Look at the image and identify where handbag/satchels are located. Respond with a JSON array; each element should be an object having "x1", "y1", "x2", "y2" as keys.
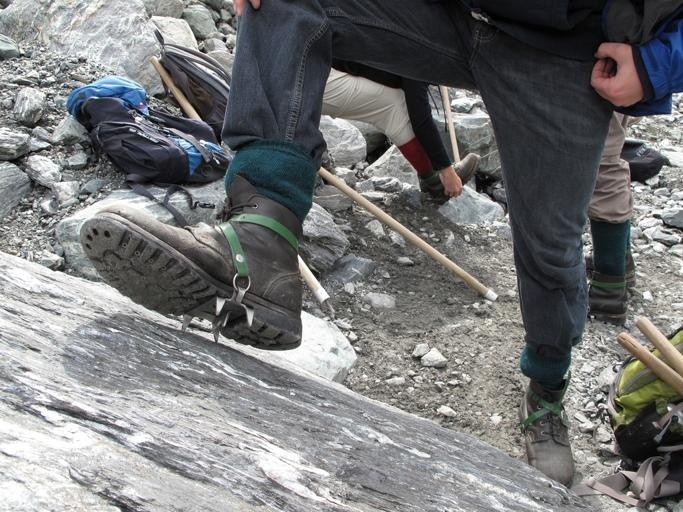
[{"x1": 620, "y1": 140, "x2": 665, "y2": 180}]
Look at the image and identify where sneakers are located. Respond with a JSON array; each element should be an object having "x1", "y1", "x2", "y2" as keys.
[{"x1": 419, "y1": 152, "x2": 481, "y2": 207}]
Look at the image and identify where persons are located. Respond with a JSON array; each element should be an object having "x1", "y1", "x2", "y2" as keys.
[
  {"x1": 79, "y1": 0, "x2": 683, "y2": 487},
  {"x1": 589, "y1": 110, "x2": 635, "y2": 326},
  {"x1": 321, "y1": 59, "x2": 482, "y2": 206}
]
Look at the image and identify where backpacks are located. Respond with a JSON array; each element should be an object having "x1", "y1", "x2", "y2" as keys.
[
  {"x1": 67, "y1": 75, "x2": 229, "y2": 186},
  {"x1": 607, "y1": 326, "x2": 683, "y2": 467},
  {"x1": 153, "y1": 29, "x2": 232, "y2": 146}
]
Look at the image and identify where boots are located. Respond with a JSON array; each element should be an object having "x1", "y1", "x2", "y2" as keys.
[
  {"x1": 585, "y1": 254, "x2": 636, "y2": 326},
  {"x1": 80, "y1": 173, "x2": 301, "y2": 350},
  {"x1": 518, "y1": 379, "x2": 573, "y2": 485}
]
